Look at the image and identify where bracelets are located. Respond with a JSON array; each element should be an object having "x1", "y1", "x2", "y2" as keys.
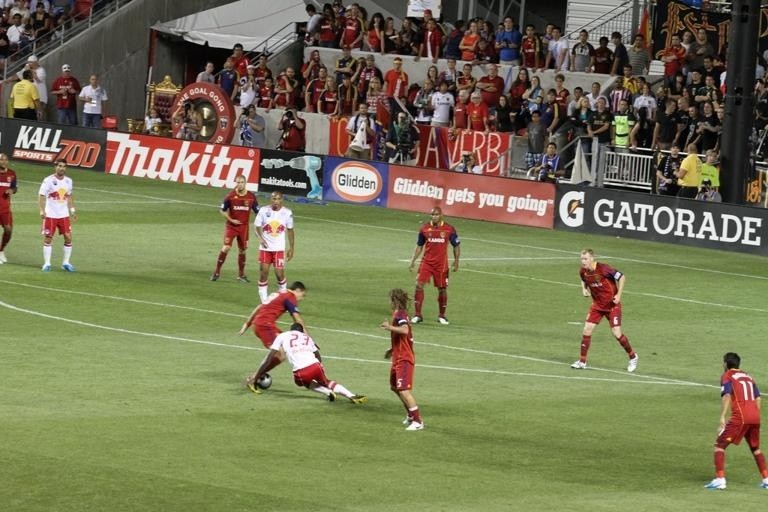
[{"x1": 70, "y1": 208, "x2": 76, "y2": 213}]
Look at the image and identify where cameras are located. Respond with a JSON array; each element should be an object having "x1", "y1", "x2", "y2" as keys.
[
  {"x1": 285, "y1": 111, "x2": 293, "y2": 119},
  {"x1": 241, "y1": 107, "x2": 250, "y2": 117},
  {"x1": 184, "y1": 103, "x2": 191, "y2": 112}
]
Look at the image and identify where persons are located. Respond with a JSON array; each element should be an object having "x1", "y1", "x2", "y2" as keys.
[
  {"x1": 0, "y1": 153, "x2": 17, "y2": 264},
  {"x1": 571, "y1": 249, "x2": 638, "y2": 372},
  {"x1": 303, "y1": 1, "x2": 497, "y2": 66},
  {"x1": 38, "y1": 158, "x2": 79, "y2": 272},
  {"x1": 704, "y1": 352, "x2": 768, "y2": 489},
  {"x1": 254, "y1": 190, "x2": 294, "y2": 304},
  {"x1": 239, "y1": 281, "x2": 306, "y2": 394},
  {"x1": 407, "y1": 206, "x2": 460, "y2": 324},
  {"x1": 233, "y1": 104, "x2": 266, "y2": 149},
  {"x1": 217, "y1": 43, "x2": 301, "y2": 114},
  {"x1": 449, "y1": 153, "x2": 482, "y2": 174},
  {"x1": 494, "y1": 67, "x2": 614, "y2": 168},
  {"x1": 1, "y1": 1, "x2": 112, "y2": 61},
  {"x1": 49, "y1": 64, "x2": 82, "y2": 125},
  {"x1": 172, "y1": 100, "x2": 204, "y2": 142},
  {"x1": 145, "y1": 109, "x2": 162, "y2": 136},
  {"x1": 79, "y1": 73, "x2": 108, "y2": 130},
  {"x1": 277, "y1": 106, "x2": 306, "y2": 153},
  {"x1": 209, "y1": 175, "x2": 259, "y2": 283},
  {"x1": 608, "y1": 27, "x2": 768, "y2": 202},
  {"x1": 386, "y1": 111, "x2": 420, "y2": 166},
  {"x1": 406, "y1": 58, "x2": 505, "y2": 132},
  {"x1": 345, "y1": 103, "x2": 376, "y2": 161},
  {"x1": 495, "y1": 17, "x2": 615, "y2": 75},
  {"x1": 196, "y1": 62, "x2": 215, "y2": 85},
  {"x1": 534, "y1": 142, "x2": 566, "y2": 183},
  {"x1": 251, "y1": 322, "x2": 368, "y2": 404},
  {"x1": 301, "y1": 44, "x2": 409, "y2": 118},
  {"x1": 2, "y1": 55, "x2": 48, "y2": 121},
  {"x1": 10, "y1": 70, "x2": 45, "y2": 121},
  {"x1": 380, "y1": 288, "x2": 424, "y2": 431}
]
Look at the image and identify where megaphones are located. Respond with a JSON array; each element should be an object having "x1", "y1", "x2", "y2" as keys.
[{"x1": 349, "y1": 117, "x2": 366, "y2": 152}]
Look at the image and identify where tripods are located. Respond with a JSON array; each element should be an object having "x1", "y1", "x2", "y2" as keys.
[{"x1": 390, "y1": 148, "x2": 403, "y2": 165}]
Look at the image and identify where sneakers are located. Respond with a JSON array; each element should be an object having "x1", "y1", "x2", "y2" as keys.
[
  {"x1": 705, "y1": 477, "x2": 726, "y2": 489},
  {"x1": 41, "y1": 265, "x2": 51, "y2": 271},
  {"x1": 61, "y1": 263, "x2": 75, "y2": 271},
  {"x1": 402, "y1": 415, "x2": 423, "y2": 430},
  {"x1": 411, "y1": 317, "x2": 450, "y2": 325},
  {"x1": 349, "y1": 394, "x2": 368, "y2": 403},
  {"x1": 0, "y1": 251, "x2": 7, "y2": 264},
  {"x1": 210, "y1": 273, "x2": 219, "y2": 281},
  {"x1": 238, "y1": 276, "x2": 248, "y2": 282},
  {"x1": 628, "y1": 353, "x2": 638, "y2": 372},
  {"x1": 246, "y1": 382, "x2": 262, "y2": 393},
  {"x1": 571, "y1": 360, "x2": 586, "y2": 369}
]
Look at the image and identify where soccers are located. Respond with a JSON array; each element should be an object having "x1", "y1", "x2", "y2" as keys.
[{"x1": 257, "y1": 374, "x2": 272, "y2": 389}]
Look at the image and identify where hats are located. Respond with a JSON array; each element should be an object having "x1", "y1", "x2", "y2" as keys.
[
  {"x1": 62, "y1": 64, "x2": 71, "y2": 71},
  {"x1": 28, "y1": 56, "x2": 37, "y2": 62}
]
